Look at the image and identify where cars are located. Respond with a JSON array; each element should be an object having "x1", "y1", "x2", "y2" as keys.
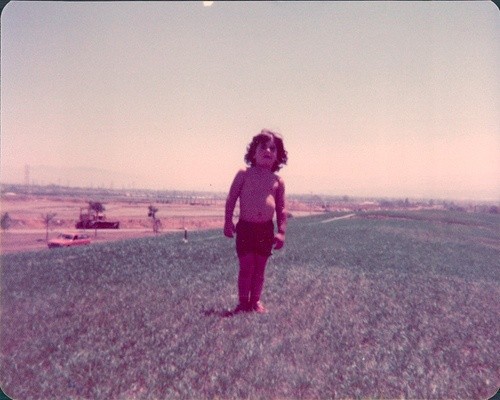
[{"x1": 47, "y1": 232, "x2": 92, "y2": 249}]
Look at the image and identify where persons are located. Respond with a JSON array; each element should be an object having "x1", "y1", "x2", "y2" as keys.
[{"x1": 224, "y1": 128, "x2": 288, "y2": 314}]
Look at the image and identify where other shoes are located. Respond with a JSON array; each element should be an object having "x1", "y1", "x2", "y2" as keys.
[
  {"x1": 249, "y1": 301, "x2": 267, "y2": 313},
  {"x1": 235, "y1": 305, "x2": 248, "y2": 313}
]
